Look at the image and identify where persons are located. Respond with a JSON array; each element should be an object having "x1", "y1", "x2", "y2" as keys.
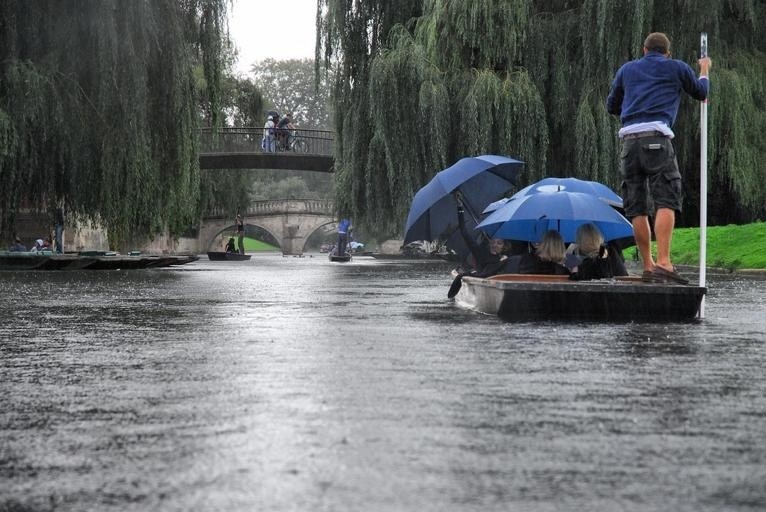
[
  {"x1": 235, "y1": 214, "x2": 245, "y2": 255},
  {"x1": 454, "y1": 190, "x2": 628, "y2": 281},
  {"x1": 336, "y1": 218, "x2": 354, "y2": 256},
  {"x1": 9, "y1": 234, "x2": 52, "y2": 252},
  {"x1": 224, "y1": 238, "x2": 236, "y2": 253},
  {"x1": 605, "y1": 32, "x2": 712, "y2": 285},
  {"x1": 261, "y1": 113, "x2": 296, "y2": 152}
]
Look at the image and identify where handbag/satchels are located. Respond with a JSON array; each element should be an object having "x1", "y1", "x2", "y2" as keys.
[{"x1": 267, "y1": 122, "x2": 274, "y2": 134}]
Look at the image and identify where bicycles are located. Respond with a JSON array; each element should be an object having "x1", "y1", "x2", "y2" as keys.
[{"x1": 268, "y1": 129, "x2": 311, "y2": 153}]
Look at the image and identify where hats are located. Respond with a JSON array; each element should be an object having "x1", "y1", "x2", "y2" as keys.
[{"x1": 268, "y1": 116, "x2": 273, "y2": 120}]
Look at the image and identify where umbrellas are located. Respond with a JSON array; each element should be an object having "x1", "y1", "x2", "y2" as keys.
[
  {"x1": 473, "y1": 177, "x2": 633, "y2": 244},
  {"x1": 403, "y1": 155, "x2": 526, "y2": 247}
]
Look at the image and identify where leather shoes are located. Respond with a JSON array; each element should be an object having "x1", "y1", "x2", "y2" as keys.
[{"x1": 642, "y1": 265, "x2": 689, "y2": 285}]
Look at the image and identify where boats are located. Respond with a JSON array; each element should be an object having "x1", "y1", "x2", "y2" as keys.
[
  {"x1": 444, "y1": 253, "x2": 709, "y2": 323},
  {"x1": 317, "y1": 240, "x2": 365, "y2": 263},
  {"x1": 0, "y1": 249, "x2": 202, "y2": 270},
  {"x1": 205, "y1": 250, "x2": 252, "y2": 261}
]
